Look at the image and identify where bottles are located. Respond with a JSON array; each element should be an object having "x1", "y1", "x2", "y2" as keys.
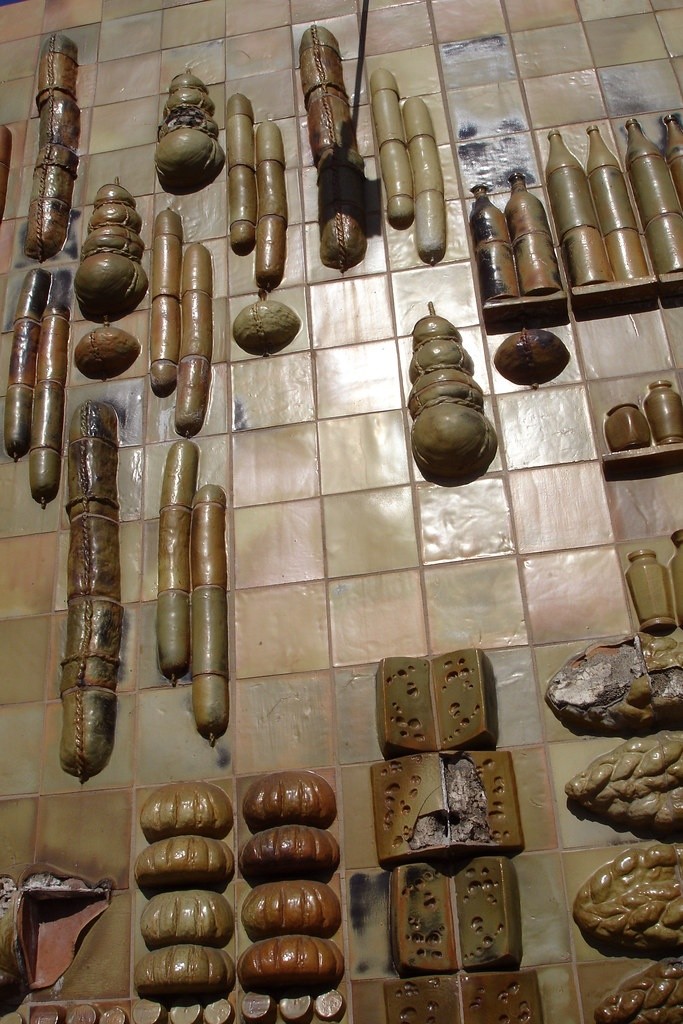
[
  {"x1": 585, "y1": 126, "x2": 648, "y2": 281},
  {"x1": 626, "y1": 549, "x2": 675, "y2": 633},
  {"x1": 669, "y1": 530, "x2": 683, "y2": 626},
  {"x1": 625, "y1": 119, "x2": 683, "y2": 274},
  {"x1": 504, "y1": 173, "x2": 562, "y2": 296},
  {"x1": 664, "y1": 116, "x2": 683, "y2": 210},
  {"x1": 643, "y1": 381, "x2": 683, "y2": 445},
  {"x1": 471, "y1": 186, "x2": 519, "y2": 301},
  {"x1": 543, "y1": 129, "x2": 612, "y2": 287}
]
